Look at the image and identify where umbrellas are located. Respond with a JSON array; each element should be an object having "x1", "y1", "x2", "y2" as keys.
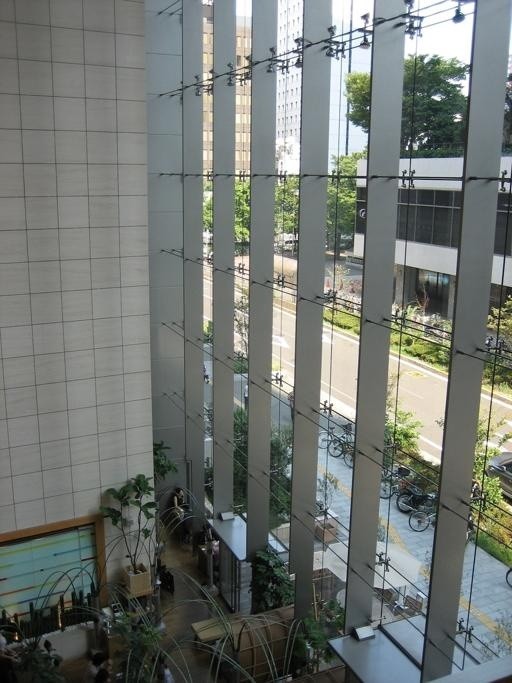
[{"x1": 325, "y1": 539, "x2": 428, "y2": 590}]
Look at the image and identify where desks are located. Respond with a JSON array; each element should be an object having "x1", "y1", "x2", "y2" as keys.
[
  {"x1": 198, "y1": 545, "x2": 206, "y2": 575},
  {"x1": 191, "y1": 612, "x2": 243, "y2": 654}
]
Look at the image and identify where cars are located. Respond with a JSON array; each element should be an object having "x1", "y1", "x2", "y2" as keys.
[{"x1": 481, "y1": 451, "x2": 512, "y2": 500}]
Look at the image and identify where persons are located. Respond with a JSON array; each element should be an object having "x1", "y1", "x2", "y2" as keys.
[
  {"x1": 287, "y1": 390, "x2": 294, "y2": 421},
  {"x1": 470, "y1": 481, "x2": 485, "y2": 500},
  {"x1": 203, "y1": 364, "x2": 210, "y2": 385},
  {"x1": 466, "y1": 513, "x2": 474, "y2": 541},
  {"x1": 173, "y1": 488, "x2": 189, "y2": 516},
  {"x1": 243, "y1": 380, "x2": 249, "y2": 412}
]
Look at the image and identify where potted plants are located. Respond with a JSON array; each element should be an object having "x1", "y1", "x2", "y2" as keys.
[{"x1": 97, "y1": 474, "x2": 157, "y2": 597}]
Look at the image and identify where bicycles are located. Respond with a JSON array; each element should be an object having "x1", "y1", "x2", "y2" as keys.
[
  {"x1": 506, "y1": 566, "x2": 512, "y2": 588},
  {"x1": 204, "y1": 374, "x2": 210, "y2": 384},
  {"x1": 318, "y1": 422, "x2": 439, "y2": 530}
]
